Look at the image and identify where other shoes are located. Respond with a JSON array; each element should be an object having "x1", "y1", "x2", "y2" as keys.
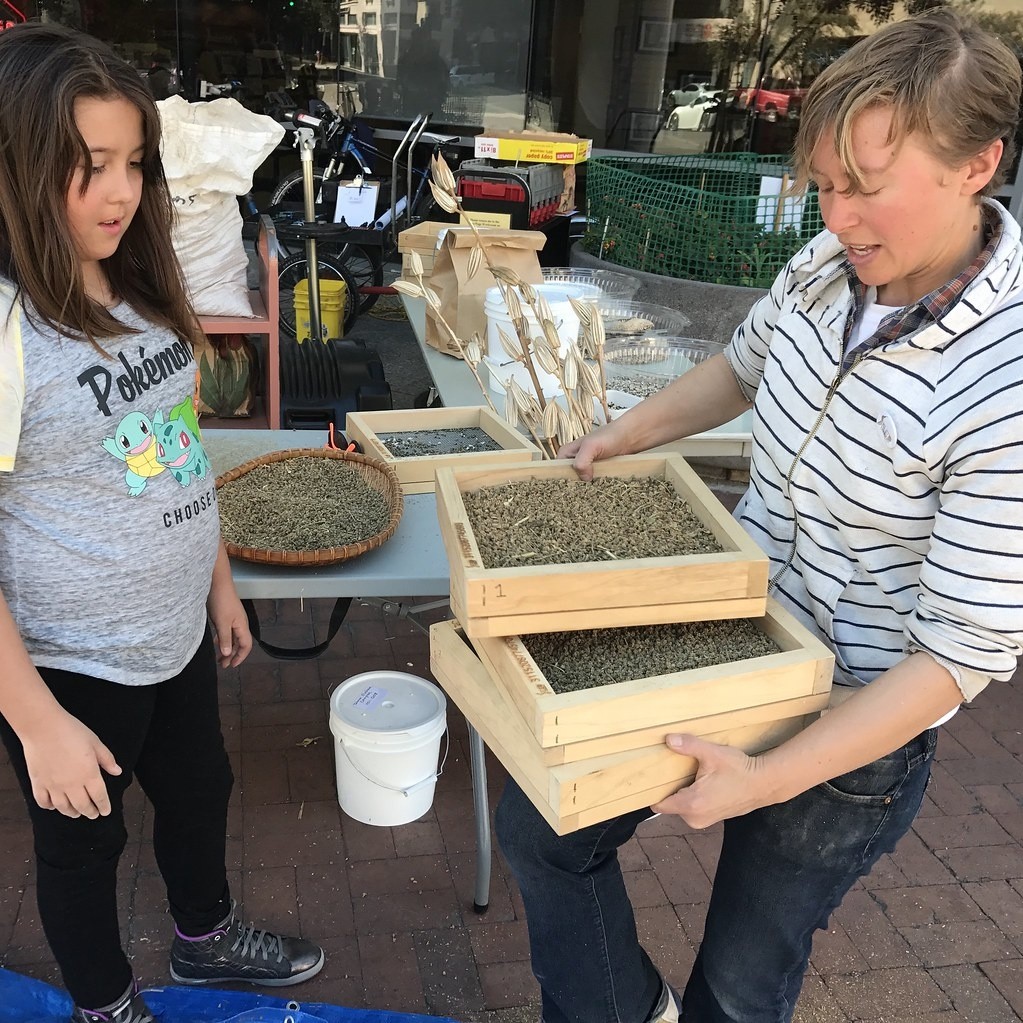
[
  {"x1": 169, "y1": 898, "x2": 325, "y2": 987},
  {"x1": 70, "y1": 963, "x2": 157, "y2": 1023}
]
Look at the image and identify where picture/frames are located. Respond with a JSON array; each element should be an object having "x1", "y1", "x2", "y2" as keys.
[
  {"x1": 624, "y1": 110, "x2": 661, "y2": 144},
  {"x1": 633, "y1": 16, "x2": 682, "y2": 56}
]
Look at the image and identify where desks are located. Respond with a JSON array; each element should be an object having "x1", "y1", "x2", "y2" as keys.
[
  {"x1": 201, "y1": 428, "x2": 493, "y2": 914},
  {"x1": 395, "y1": 285, "x2": 756, "y2": 461}
]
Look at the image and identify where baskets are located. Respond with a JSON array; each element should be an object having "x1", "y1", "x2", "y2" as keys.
[{"x1": 213, "y1": 447, "x2": 404, "y2": 567}]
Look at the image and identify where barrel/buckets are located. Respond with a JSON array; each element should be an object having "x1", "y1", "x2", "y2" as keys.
[
  {"x1": 483, "y1": 285, "x2": 584, "y2": 398},
  {"x1": 328, "y1": 671, "x2": 448, "y2": 827},
  {"x1": 293, "y1": 279, "x2": 347, "y2": 345}
]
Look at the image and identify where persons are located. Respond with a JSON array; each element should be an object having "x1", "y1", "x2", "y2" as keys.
[
  {"x1": 494, "y1": 14, "x2": 1023, "y2": 1023},
  {"x1": 0, "y1": 23, "x2": 325, "y2": 1023}
]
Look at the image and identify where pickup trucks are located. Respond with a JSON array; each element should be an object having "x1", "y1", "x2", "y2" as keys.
[{"x1": 745, "y1": 74, "x2": 811, "y2": 124}]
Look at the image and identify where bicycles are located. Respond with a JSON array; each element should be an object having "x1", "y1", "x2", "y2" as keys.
[{"x1": 241, "y1": 79, "x2": 580, "y2": 341}]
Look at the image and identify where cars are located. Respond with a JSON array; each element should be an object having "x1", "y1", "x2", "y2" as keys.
[
  {"x1": 448, "y1": 63, "x2": 498, "y2": 87},
  {"x1": 665, "y1": 90, "x2": 747, "y2": 133},
  {"x1": 666, "y1": 82, "x2": 724, "y2": 108}
]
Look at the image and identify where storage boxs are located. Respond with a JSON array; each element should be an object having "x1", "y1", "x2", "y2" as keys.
[
  {"x1": 401, "y1": 274, "x2": 430, "y2": 287},
  {"x1": 343, "y1": 405, "x2": 543, "y2": 496},
  {"x1": 395, "y1": 222, "x2": 466, "y2": 254},
  {"x1": 432, "y1": 452, "x2": 769, "y2": 637},
  {"x1": 472, "y1": 129, "x2": 593, "y2": 164},
  {"x1": 402, "y1": 255, "x2": 433, "y2": 277},
  {"x1": 428, "y1": 619, "x2": 821, "y2": 836},
  {"x1": 454, "y1": 157, "x2": 565, "y2": 207},
  {"x1": 293, "y1": 279, "x2": 347, "y2": 345},
  {"x1": 450, "y1": 599, "x2": 835, "y2": 767},
  {"x1": 458, "y1": 177, "x2": 560, "y2": 226}
]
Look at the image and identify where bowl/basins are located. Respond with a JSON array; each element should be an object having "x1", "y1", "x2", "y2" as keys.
[
  {"x1": 541, "y1": 267, "x2": 640, "y2": 301},
  {"x1": 595, "y1": 336, "x2": 729, "y2": 400},
  {"x1": 578, "y1": 300, "x2": 690, "y2": 365},
  {"x1": 592, "y1": 390, "x2": 644, "y2": 424}
]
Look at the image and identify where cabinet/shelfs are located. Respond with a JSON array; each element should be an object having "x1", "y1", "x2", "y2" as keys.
[{"x1": 190, "y1": 212, "x2": 280, "y2": 430}]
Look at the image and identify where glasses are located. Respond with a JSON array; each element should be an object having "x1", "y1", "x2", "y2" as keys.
[{"x1": 326, "y1": 423, "x2": 362, "y2": 453}]
